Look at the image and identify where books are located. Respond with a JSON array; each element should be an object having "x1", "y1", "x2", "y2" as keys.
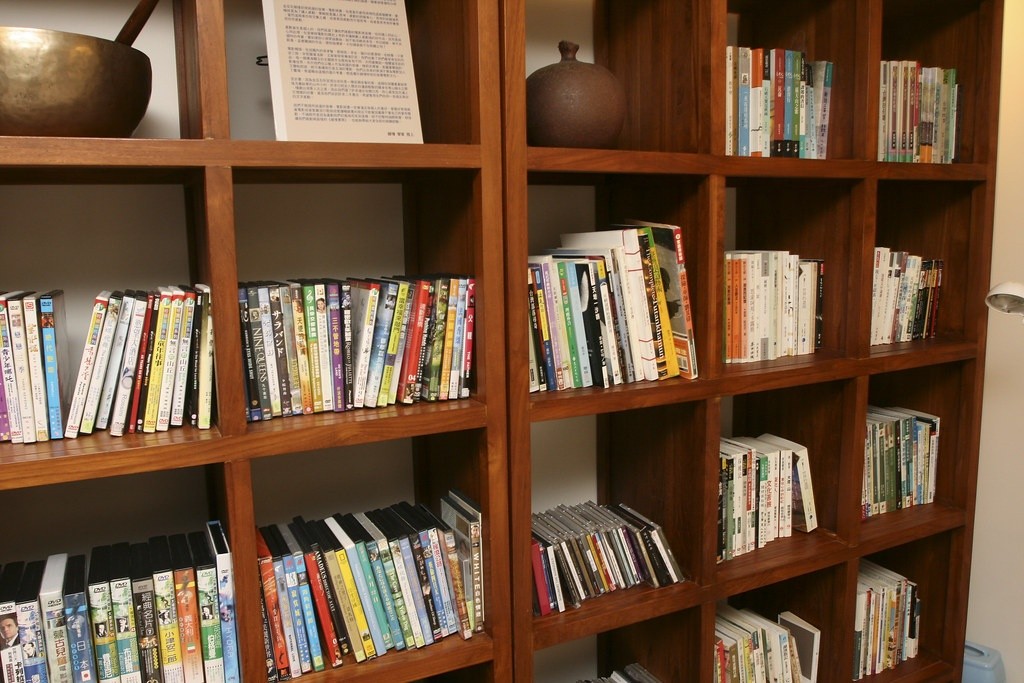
[
  {"x1": 722, "y1": 250, "x2": 825, "y2": 363},
  {"x1": 530, "y1": 218, "x2": 698, "y2": 392},
  {"x1": 576, "y1": 663, "x2": 663, "y2": 683},
  {"x1": 716, "y1": 433, "x2": 817, "y2": 563},
  {"x1": 878, "y1": 61, "x2": 958, "y2": 163},
  {"x1": 531, "y1": 500, "x2": 684, "y2": 616},
  {"x1": 0, "y1": 520, "x2": 241, "y2": 683},
  {"x1": 0, "y1": 284, "x2": 212, "y2": 444},
  {"x1": 255, "y1": 489, "x2": 485, "y2": 683},
  {"x1": 860, "y1": 406, "x2": 940, "y2": 519},
  {"x1": 870, "y1": 247, "x2": 944, "y2": 346},
  {"x1": 237, "y1": 274, "x2": 476, "y2": 422},
  {"x1": 714, "y1": 602, "x2": 820, "y2": 683},
  {"x1": 726, "y1": 46, "x2": 833, "y2": 159},
  {"x1": 852, "y1": 562, "x2": 921, "y2": 681}
]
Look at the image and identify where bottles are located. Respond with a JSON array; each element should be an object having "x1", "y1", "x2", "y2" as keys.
[{"x1": 526, "y1": 38, "x2": 627, "y2": 148}]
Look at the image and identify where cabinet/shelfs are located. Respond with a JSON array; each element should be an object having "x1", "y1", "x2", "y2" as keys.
[{"x1": 1, "y1": 0, "x2": 1002, "y2": 683}]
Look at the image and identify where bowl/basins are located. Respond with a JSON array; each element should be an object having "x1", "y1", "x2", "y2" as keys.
[{"x1": 0, "y1": 27, "x2": 152, "y2": 138}]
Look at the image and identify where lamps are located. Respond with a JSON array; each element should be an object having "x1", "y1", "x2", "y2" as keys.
[{"x1": 984, "y1": 282, "x2": 1024, "y2": 316}]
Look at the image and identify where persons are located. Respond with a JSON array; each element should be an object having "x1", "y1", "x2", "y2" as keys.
[
  {"x1": 471, "y1": 525, "x2": 479, "y2": 538},
  {"x1": 293, "y1": 299, "x2": 302, "y2": 312},
  {"x1": 317, "y1": 298, "x2": 325, "y2": 312},
  {"x1": 265, "y1": 658, "x2": 276, "y2": 677},
  {"x1": 0, "y1": 616, "x2": 20, "y2": 649}
]
